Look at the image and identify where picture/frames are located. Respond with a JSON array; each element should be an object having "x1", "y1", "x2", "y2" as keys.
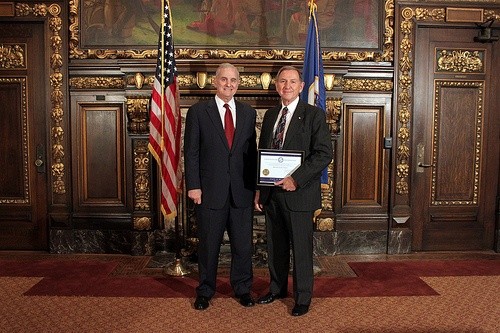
[
  {"x1": 256, "y1": 149, "x2": 305, "y2": 187},
  {"x1": 69, "y1": 0, "x2": 395, "y2": 60}
]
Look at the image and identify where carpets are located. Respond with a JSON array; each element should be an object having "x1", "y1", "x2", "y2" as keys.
[{"x1": 0, "y1": 253, "x2": 500, "y2": 297}]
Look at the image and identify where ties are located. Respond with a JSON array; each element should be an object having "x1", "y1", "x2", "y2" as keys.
[
  {"x1": 273, "y1": 107, "x2": 289, "y2": 150},
  {"x1": 223, "y1": 103, "x2": 235, "y2": 151}
]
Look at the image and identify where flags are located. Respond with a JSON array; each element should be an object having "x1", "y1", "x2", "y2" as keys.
[
  {"x1": 302, "y1": 0, "x2": 329, "y2": 185},
  {"x1": 148, "y1": 0, "x2": 183, "y2": 222}
]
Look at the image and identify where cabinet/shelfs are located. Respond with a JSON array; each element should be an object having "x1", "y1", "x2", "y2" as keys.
[
  {"x1": 69, "y1": 89, "x2": 133, "y2": 218},
  {"x1": 334, "y1": 91, "x2": 392, "y2": 216}
]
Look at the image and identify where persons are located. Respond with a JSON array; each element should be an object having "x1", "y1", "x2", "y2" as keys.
[
  {"x1": 253, "y1": 65, "x2": 334, "y2": 316},
  {"x1": 182, "y1": 62, "x2": 259, "y2": 311}
]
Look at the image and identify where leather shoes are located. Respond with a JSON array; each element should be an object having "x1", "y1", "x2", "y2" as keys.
[
  {"x1": 194, "y1": 296, "x2": 209, "y2": 309},
  {"x1": 257, "y1": 292, "x2": 288, "y2": 304},
  {"x1": 234, "y1": 294, "x2": 254, "y2": 306},
  {"x1": 292, "y1": 300, "x2": 311, "y2": 316}
]
